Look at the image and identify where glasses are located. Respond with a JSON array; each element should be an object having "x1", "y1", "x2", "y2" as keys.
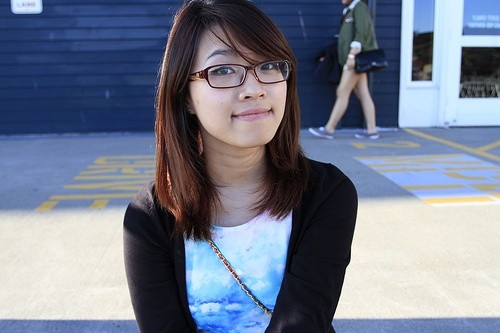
[{"x1": 190, "y1": 59, "x2": 292, "y2": 89}]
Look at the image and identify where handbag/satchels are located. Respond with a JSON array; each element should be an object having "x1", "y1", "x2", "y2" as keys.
[{"x1": 353, "y1": 48, "x2": 388, "y2": 74}]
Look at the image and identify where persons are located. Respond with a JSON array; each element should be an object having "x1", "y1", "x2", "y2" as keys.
[
  {"x1": 122, "y1": 0, "x2": 360, "y2": 333},
  {"x1": 308, "y1": 0, "x2": 381, "y2": 140}
]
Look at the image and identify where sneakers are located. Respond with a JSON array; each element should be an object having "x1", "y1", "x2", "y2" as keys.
[
  {"x1": 309, "y1": 127, "x2": 334, "y2": 139},
  {"x1": 354, "y1": 131, "x2": 381, "y2": 139}
]
